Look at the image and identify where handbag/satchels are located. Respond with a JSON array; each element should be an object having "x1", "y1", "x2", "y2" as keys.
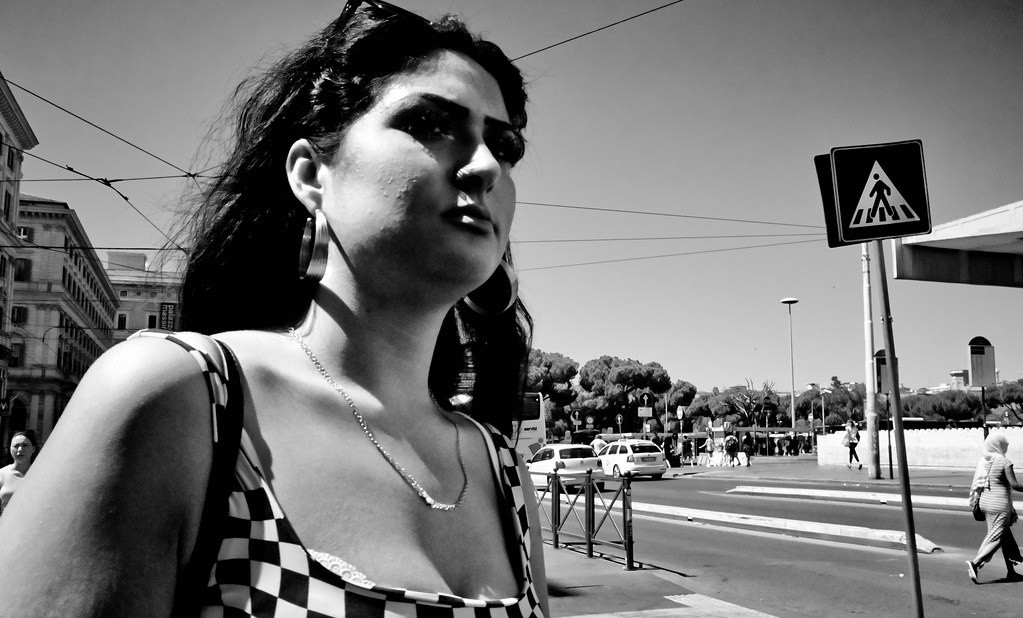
[
  {"x1": 972, "y1": 501, "x2": 986, "y2": 522},
  {"x1": 842, "y1": 427, "x2": 850, "y2": 447},
  {"x1": 856, "y1": 433, "x2": 860, "y2": 440},
  {"x1": 743, "y1": 444, "x2": 749, "y2": 452}
]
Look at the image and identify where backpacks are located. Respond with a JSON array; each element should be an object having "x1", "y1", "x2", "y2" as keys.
[{"x1": 725, "y1": 438, "x2": 738, "y2": 452}]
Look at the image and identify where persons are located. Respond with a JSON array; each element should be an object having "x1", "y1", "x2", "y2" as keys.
[
  {"x1": 742, "y1": 431, "x2": 753, "y2": 466},
  {"x1": 590, "y1": 435, "x2": 607, "y2": 455},
  {"x1": 642, "y1": 432, "x2": 695, "y2": 468},
  {"x1": 965, "y1": 433, "x2": 1023, "y2": 584},
  {"x1": 753, "y1": 436, "x2": 812, "y2": 456},
  {"x1": 844, "y1": 419, "x2": 863, "y2": 469},
  {"x1": 725, "y1": 432, "x2": 741, "y2": 467},
  {"x1": 0, "y1": 431, "x2": 38, "y2": 518},
  {"x1": 699, "y1": 433, "x2": 714, "y2": 468},
  {"x1": 1, "y1": 0, "x2": 550, "y2": 618}
]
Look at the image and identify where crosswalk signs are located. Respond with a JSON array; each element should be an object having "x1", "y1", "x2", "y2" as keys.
[{"x1": 830, "y1": 139, "x2": 932, "y2": 244}]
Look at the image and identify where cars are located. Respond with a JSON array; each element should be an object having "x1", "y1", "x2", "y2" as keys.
[
  {"x1": 524, "y1": 443, "x2": 605, "y2": 493},
  {"x1": 597, "y1": 438, "x2": 668, "y2": 481}
]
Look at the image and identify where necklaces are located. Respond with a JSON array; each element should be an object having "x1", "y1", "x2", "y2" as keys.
[{"x1": 285, "y1": 324, "x2": 469, "y2": 511}]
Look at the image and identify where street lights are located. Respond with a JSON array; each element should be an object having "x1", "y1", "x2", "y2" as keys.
[{"x1": 779, "y1": 297, "x2": 800, "y2": 433}]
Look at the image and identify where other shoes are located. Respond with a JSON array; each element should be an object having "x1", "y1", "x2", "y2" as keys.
[
  {"x1": 1006, "y1": 571, "x2": 1023, "y2": 581},
  {"x1": 745, "y1": 464, "x2": 750, "y2": 467},
  {"x1": 736, "y1": 463, "x2": 740, "y2": 466},
  {"x1": 847, "y1": 465, "x2": 852, "y2": 468},
  {"x1": 858, "y1": 464, "x2": 863, "y2": 470},
  {"x1": 965, "y1": 560, "x2": 980, "y2": 584}
]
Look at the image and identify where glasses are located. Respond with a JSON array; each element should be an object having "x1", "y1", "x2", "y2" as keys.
[{"x1": 332, "y1": 0, "x2": 442, "y2": 37}]
